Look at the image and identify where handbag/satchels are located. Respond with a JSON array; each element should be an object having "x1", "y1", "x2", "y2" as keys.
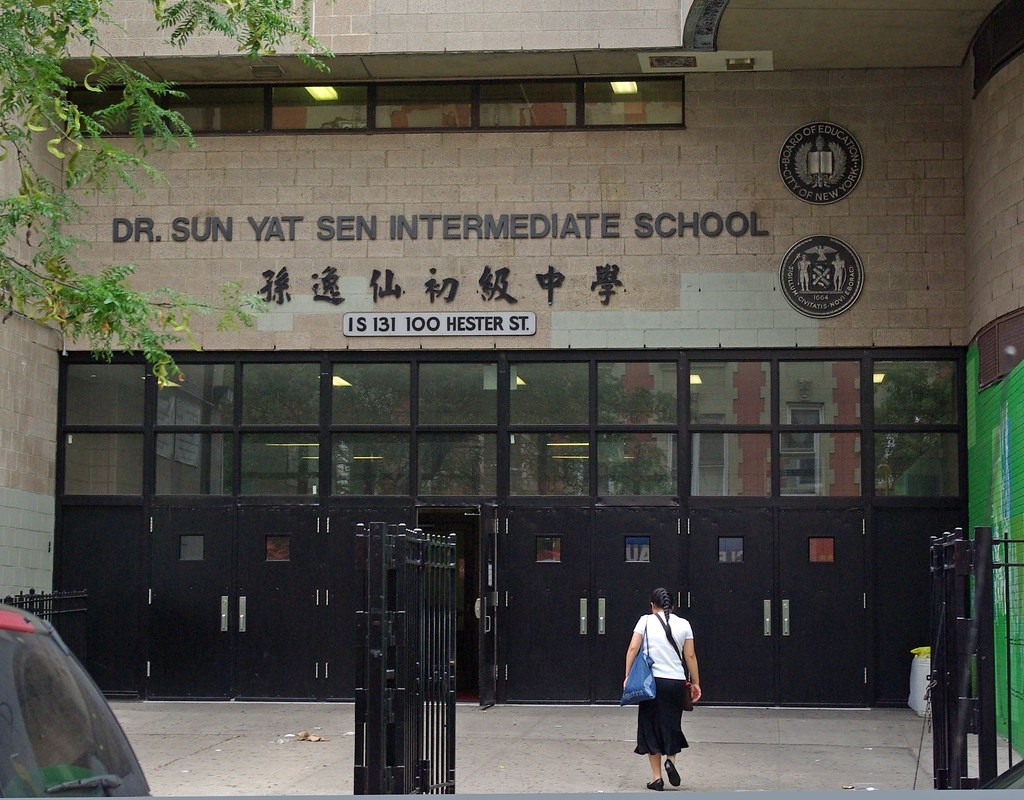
[
  {"x1": 620, "y1": 652, "x2": 655, "y2": 706},
  {"x1": 684, "y1": 684, "x2": 694, "y2": 712}
]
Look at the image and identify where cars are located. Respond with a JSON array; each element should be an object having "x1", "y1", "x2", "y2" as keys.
[{"x1": 0, "y1": 603, "x2": 150, "y2": 799}]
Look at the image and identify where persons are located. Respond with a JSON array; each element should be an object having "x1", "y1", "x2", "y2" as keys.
[{"x1": 623, "y1": 588, "x2": 701, "y2": 791}]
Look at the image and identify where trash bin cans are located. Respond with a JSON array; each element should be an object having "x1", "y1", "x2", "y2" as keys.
[{"x1": 907, "y1": 646, "x2": 932, "y2": 718}]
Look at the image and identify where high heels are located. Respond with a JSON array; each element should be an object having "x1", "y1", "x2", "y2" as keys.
[
  {"x1": 664, "y1": 759, "x2": 681, "y2": 786},
  {"x1": 647, "y1": 778, "x2": 664, "y2": 791}
]
